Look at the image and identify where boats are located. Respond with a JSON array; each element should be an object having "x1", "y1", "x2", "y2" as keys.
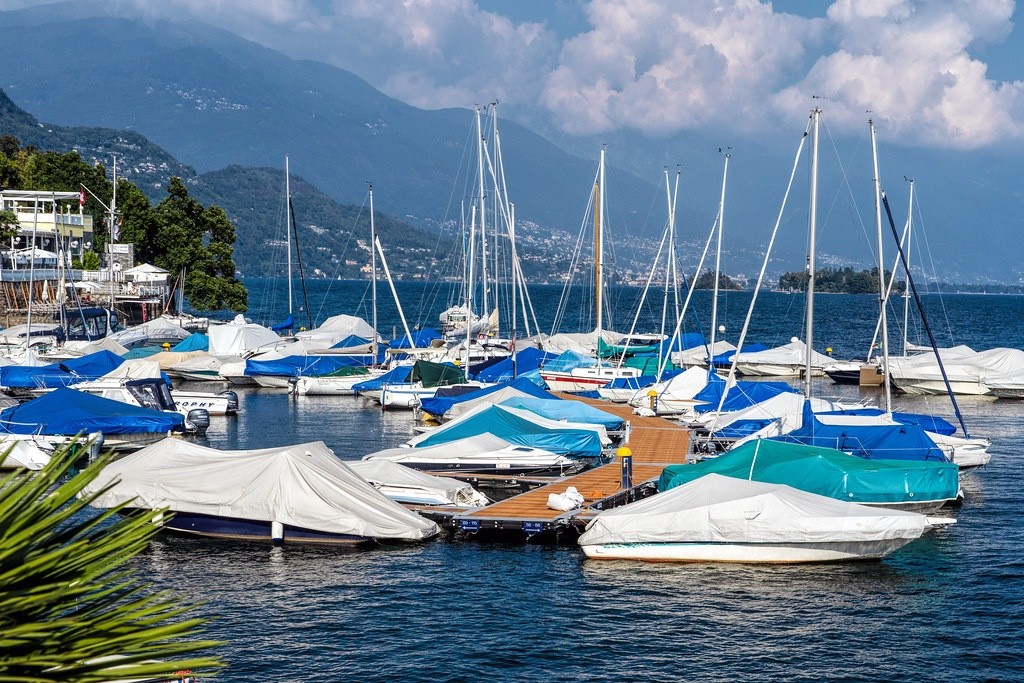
[
  {"x1": 0, "y1": 102, "x2": 993, "y2": 547},
  {"x1": 73, "y1": 435, "x2": 440, "y2": 547},
  {"x1": 656, "y1": 436, "x2": 966, "y2": 515},
  {"x1": 732, "y1": 409, "x2": 992, "y2": 471},
  {"x1": 0, "y1": 386, "x2": 186, "y2": 475},
  {"x1": 881, "y1": 343, "x2": 1024, "y2": 400},
  {"x1": 576, "y1": 472, "x2": 959, "y2": 563},
  {"x1": 357, "y1": 433, "x2": 584, "y2": 489},
  {"x1": 340, "y1": 455, "x2": 487, "y2": 527}
]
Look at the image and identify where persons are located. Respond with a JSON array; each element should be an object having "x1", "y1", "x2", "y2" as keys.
[
  {"x1": 54, "y1": 326, "x2": 67, "y2": 348},
  {"x1": 76, "y1": 290, "x2": 92, "y2": 303}
]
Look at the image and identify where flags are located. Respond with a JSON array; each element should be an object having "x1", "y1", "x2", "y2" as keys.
[{"x1": 80, "y1": 186, "x2": 87, "y2": 205}]
